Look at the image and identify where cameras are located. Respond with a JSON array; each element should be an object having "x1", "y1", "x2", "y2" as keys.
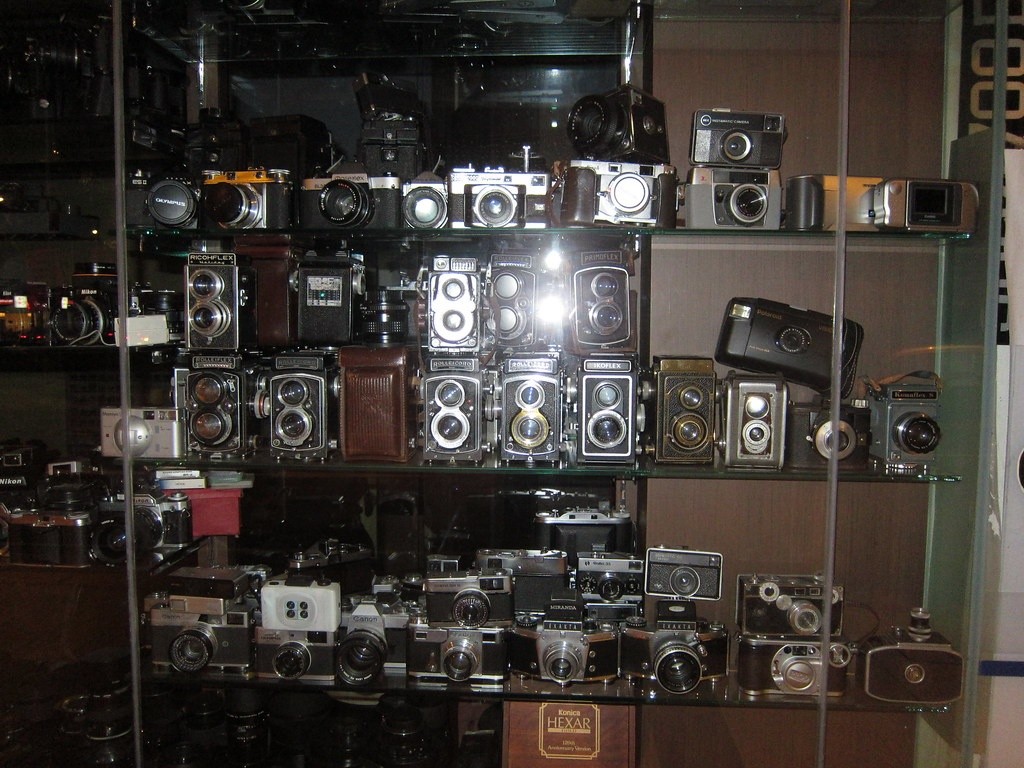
[{"x1": 0, "y1": 83, "x2": 980, "y2": 768}]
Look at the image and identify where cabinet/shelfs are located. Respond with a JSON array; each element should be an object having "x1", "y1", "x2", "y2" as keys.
[{"x1": 0, "y1": 0, "x2": 1010, "y2": 767}]
